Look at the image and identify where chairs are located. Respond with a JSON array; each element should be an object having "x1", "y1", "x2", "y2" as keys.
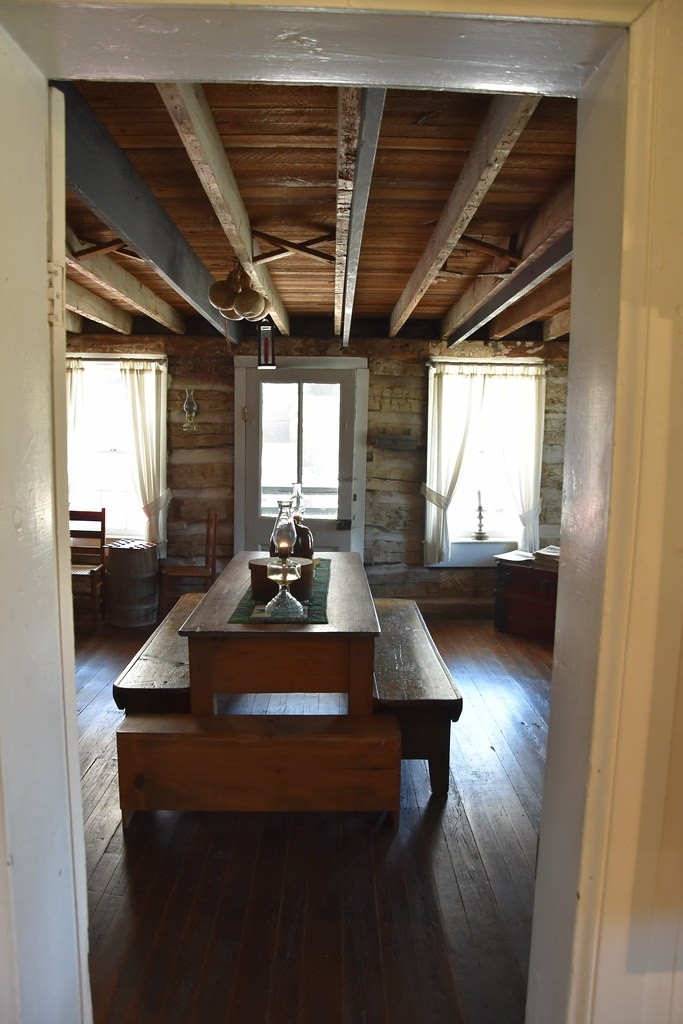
[{"x1": 69, "y1": 507, "x2": 106, "y2": 620}]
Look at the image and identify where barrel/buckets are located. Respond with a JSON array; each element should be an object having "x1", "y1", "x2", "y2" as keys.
[{"x1": 106, "y1": 548, "x2": 158, "y2": 627}]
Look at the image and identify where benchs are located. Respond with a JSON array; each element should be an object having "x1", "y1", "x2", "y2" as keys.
[
  {"x1": 110, "y1": 592, "x2": 206, "y2": 709},
  {"x1": 370, "y1": 598, "x2": 463, "y2": 799}
]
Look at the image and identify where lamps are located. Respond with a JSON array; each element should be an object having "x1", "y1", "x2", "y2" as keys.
[
  {"x1": 267, "y1": 498, "x2": 304, "y2": 614},
  {"x1": 182, "y1": 388, "x2": 200, "y2": 431}
]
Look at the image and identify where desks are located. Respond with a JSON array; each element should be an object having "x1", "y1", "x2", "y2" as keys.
[
  {"x1": 177, "y1": 551, "x2": 382, "y2": 730},
  {"x1": 495, "y1": 561, "x2": 559, "y2": 644}
]
